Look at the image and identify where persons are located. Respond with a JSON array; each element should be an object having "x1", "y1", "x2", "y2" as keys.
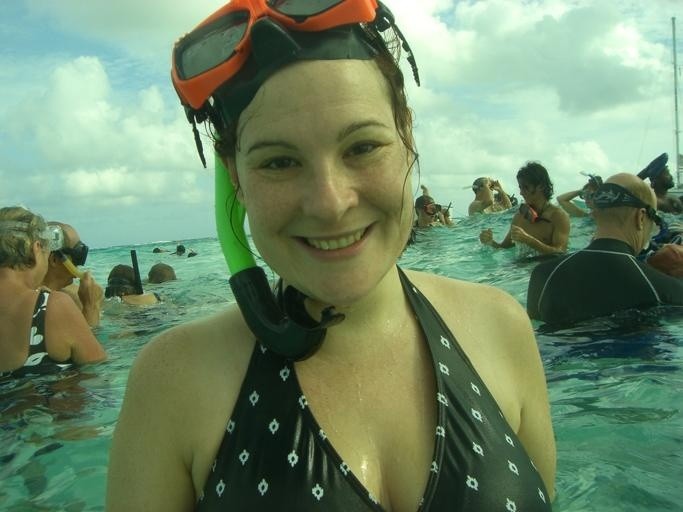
[
  {"x1": 0, "y1": 206, "x2": 198, "y2": 383},
  {"x1": 462, "y1": 177, "x2": 512, "y2": 218},
  {"x1": 479, "y1": 162, "x2": 570, "y2": 259},
  {"x1": 106, "y1": 0, "x2": 556, "y2": 512},
  {"x1": 415, "y1": 184, "x2": 455, "y2": 228},
  {"x1": 526, "y1": 152, "x2": 683, "y2": 323}
]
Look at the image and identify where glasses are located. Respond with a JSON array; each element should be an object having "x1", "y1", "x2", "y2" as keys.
[
  {"x1": 651, "y1": 215, "x2": 670, "y2": 241},
  {"x1": 170, "y1": 0, "x2": 383, "y2": 113},
  {"x1": 423, "y1": 204, "x2": 441, "y2": 216},
  {"x1": 44, "y1": 223, "x2": 64, "y2": 253},
  {"x1": 70, "y1": 241, "x2": 89, "y2": 267}
]
